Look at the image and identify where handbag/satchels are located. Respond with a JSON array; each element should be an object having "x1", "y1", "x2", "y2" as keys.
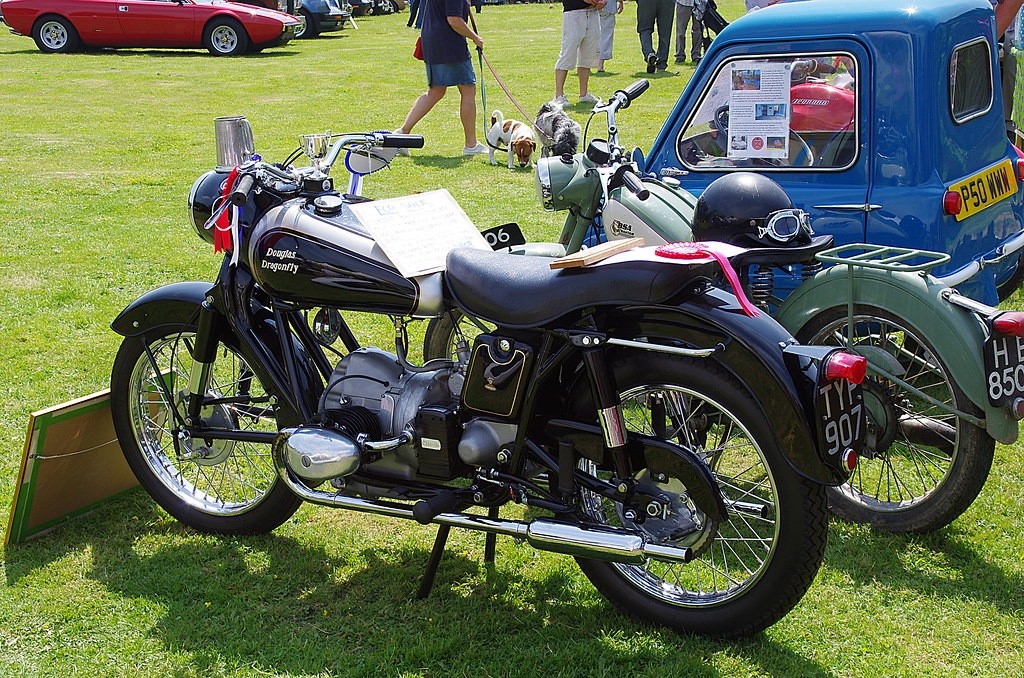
[{"x1": 413, "y1": 37, "x2": 424, "y2": 60}]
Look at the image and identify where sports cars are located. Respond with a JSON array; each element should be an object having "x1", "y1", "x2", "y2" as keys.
[{"x1": 0, "y1": 0, "x2": 307, "y2": 57}]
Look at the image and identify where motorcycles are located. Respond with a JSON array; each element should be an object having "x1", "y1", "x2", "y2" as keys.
[
  {"x1": 531, "y1": 80, "x2": 1023, "y2": 538},
  {"x1": 108, "y1": 129, "x2": 866, "y2": 642}
]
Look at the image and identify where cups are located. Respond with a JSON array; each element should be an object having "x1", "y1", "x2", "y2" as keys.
[
  {"x1": 298, "y1": 129, "x2": 331, "y2": 168},
  {"x1": 213, "y1": 114, "x2": 255, "y2": 167}
]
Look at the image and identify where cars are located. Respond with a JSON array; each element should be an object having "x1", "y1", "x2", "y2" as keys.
[
  {"x1": 575, "y1": 0, "x2": 1023, "y2": 366},
  {"x1": 225, "y1": 0, "x2": 409, "y2": 40}
]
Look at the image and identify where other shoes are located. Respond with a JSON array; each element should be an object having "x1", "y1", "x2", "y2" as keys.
[
  {"x1": 596, "y1": 62, "x2": 605, "y2": 73},
  {"x1": 675, "y1": 58, "x2": 683, "y2": 62},
  {"x1": 692, "y1": 58, "x2": 699, "y2": 62},
  {"x1": 647, "y1": 53, "x2": 657, "y2": 74},
  {"x1": 657, "y1": 67, "x2": 665, "y2": 72},
  {"x1": 463, "y1": 140, "x2": 498, "y2": 155},
  {"x1": 391, "y1": 129, "x2": 411, "y2": 157}
]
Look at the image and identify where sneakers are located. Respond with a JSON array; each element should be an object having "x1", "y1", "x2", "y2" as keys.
[
  {"x1": 579, "y1": 92, "x2": 599, "y2": 103},
  {"x1": 554, "y1": 95, "x2": 572, "y2": 108}
]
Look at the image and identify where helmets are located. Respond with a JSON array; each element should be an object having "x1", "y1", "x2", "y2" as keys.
[{"x1": 691, "y1": 172, "x2": 812, "y2": 247}]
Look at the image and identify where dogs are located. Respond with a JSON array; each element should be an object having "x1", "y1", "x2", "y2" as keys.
[{"x1": 487, "y1": 110, "x2": 537, "y2": 169}]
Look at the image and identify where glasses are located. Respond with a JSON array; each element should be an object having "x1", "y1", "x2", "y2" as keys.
[{"x1": 764, "y1": 208, "x2": 815, "y2": 243}]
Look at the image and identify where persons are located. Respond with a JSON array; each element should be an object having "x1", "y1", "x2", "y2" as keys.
[
  {"x1": 674, "y1": 0, "x2": 709, "y2": 62},
  {"x1": 551, "y1": 0, "x2": 608, "y2": 107},
  {"x1": 634, "y1": 0, "x2": 676, "y2": 73},
  {"x1": 996, "y1": 0, "x2": 1024, "y2": 49},
  {"x1": 745, "y1": 0, "x2": 823, "y2": 83},
  {"x1": 393, "y1": 0, "x2": 497, "y2": 156},
  {"x1": 597, "y1": 0, "x2": 623, "y2": 72}
]
